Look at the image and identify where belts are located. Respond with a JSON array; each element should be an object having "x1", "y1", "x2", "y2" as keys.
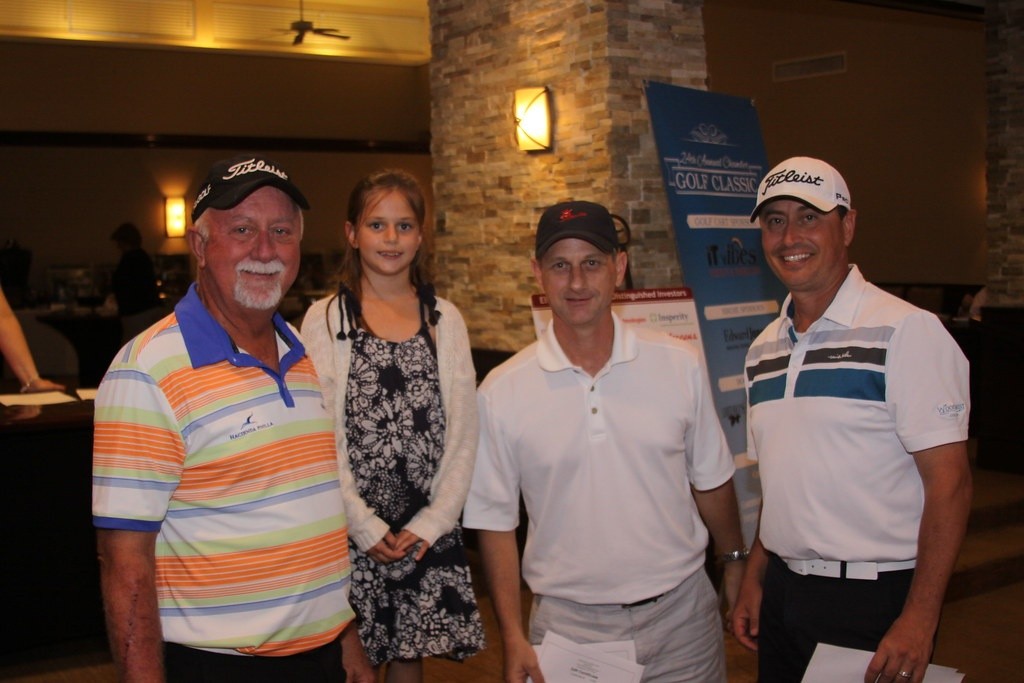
[{"x1": 781, "y1": 557, "x2": 917, "y2": 581}]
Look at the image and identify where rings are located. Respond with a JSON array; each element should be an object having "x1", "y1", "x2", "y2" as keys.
[{"x1": 897, "y1": 671, "x2": 910, "y2": 679}]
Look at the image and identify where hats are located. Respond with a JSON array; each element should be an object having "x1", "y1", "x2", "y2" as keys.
[
  {"x1": 535, "y1": 200, "x2": 619, "y2": 262},
  {"x1": 190, "y1": 152, "x2": 310, "y2": 226},
  {"x1": 749, "y1": 156, "x2": 851, "y2": 224}
]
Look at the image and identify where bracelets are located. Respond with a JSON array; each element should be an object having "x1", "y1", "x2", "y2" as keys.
[
  {"x1": 721, "y1": 548, "x2": 749, "y2": 562},
  {"x1": 20, "y1": 372, "x2": 40, "y2": 393}
]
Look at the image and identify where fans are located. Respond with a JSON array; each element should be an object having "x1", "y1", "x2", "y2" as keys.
[{"x1": 270, "y1": 0, "x2": 350, "y2": 45}]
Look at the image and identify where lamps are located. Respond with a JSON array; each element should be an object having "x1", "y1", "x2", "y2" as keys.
[{"x1": 510, "y1": 87, "x2": 551, "y2": 152}]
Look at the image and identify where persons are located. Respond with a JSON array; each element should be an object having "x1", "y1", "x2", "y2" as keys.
[
  {"x1": 92, "y1": 154, "x2": 376, "y2": 683},
  {"x1": 462, "y1": 201, "x2": 749, "y2": 683},
  {"x1": 727, "y1": 156, "x2": 970, "y2": 682},
  {"x1": 101, "y1": 223, "x2": 158, "y2": 342},
  {"x1": 299, "y1": 168, "x2": 479, "y2": 683},
  {"x1": 0, "y1": 285, "x2": 66, "y2": 394}
]
